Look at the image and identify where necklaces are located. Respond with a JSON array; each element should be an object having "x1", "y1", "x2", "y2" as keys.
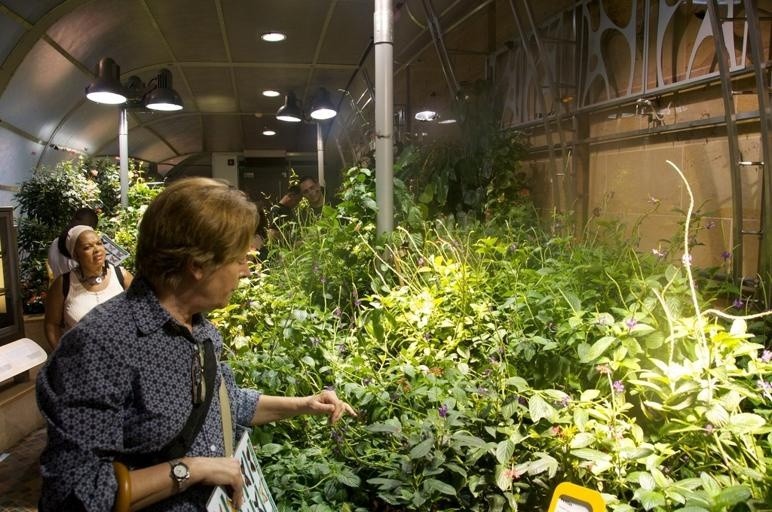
[{"x1": 77, "y1": 261, "x2": 112, "y2": 285}]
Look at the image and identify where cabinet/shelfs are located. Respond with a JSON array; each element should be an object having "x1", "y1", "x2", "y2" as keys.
[{"x1": 1, "y1": 205, "x2": 31, "y2": 385}]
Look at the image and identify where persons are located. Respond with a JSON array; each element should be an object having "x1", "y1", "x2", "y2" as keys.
[
  {"x1": 297, "y1": 177, "x2": 325, "y2": 228},
  {"x1": 269, "y1": 186, "x2": 301, "y2": 240},
  {"x1": 44, "y1": 225, "x2": 135, "y2": 351},
  {"x1": 49, "y1": 208, "x2": 98, "y2": 279},
  {"x1": 35, "y1": 175, "x2": 356, "y2": 511}
]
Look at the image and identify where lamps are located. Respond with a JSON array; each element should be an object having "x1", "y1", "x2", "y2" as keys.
[
  {"x1": 77, "y1": 54, "x2": 187, "y2": 116},
  {"x1": 269, "y1": 87, "x2": 344, "y2": 130}
]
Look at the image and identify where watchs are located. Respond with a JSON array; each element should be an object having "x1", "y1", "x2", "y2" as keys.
[{"x1": 172, "y1": 458, "x2": 190, "y2": 493}]
